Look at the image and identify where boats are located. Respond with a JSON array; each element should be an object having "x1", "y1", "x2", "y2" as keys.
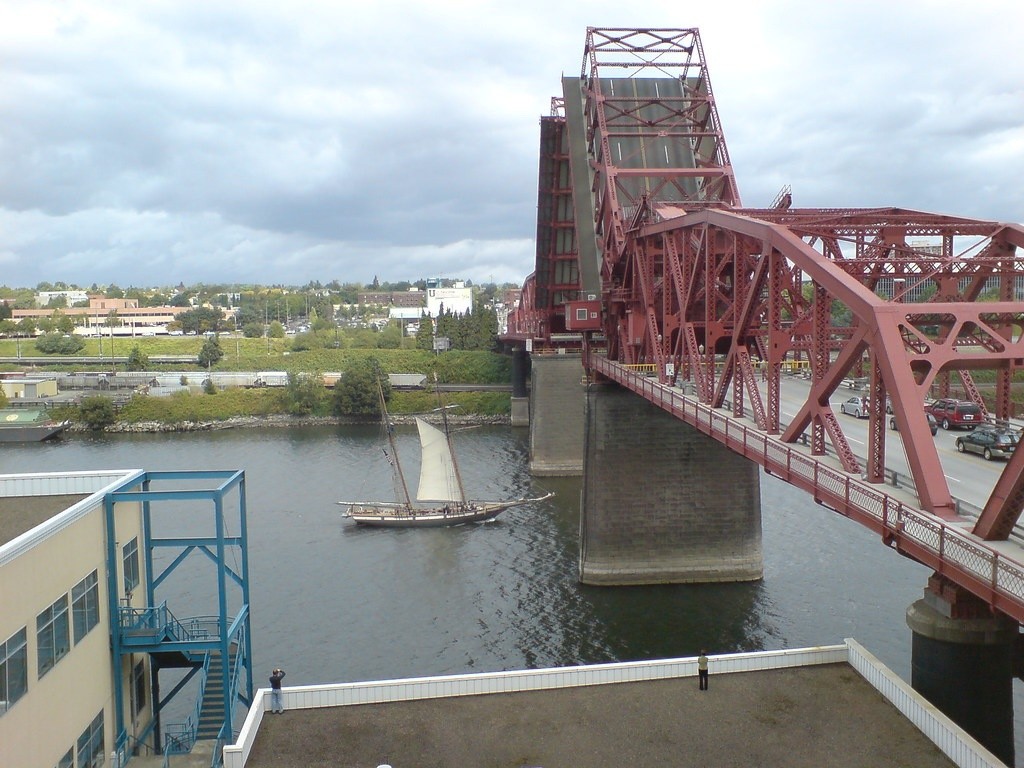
[{"x1": 0, "y1": 410, "x2": 74, "y2": 442}]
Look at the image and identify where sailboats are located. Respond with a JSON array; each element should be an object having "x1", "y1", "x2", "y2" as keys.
[{"x1": 337, "y1": 373, "x2": 554, "y2": 529}]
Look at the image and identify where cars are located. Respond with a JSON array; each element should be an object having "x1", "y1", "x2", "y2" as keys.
[
  {"x1": 886, "y1": 398, "x2": 893, "y2": 414},
  {"x1": 954, "y1": 428, "x2": 1021, "y2": 462},
  {"x1": 888, "y1": 411, "x2": 938, "y2": 436},
  {"x1": 840, "y1": 396, "x2": 881, "y2": 420}
]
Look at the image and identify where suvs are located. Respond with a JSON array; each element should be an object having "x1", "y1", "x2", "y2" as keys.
[{"x1": 923, "y1": 398, "x2": 983, "y2": 432}]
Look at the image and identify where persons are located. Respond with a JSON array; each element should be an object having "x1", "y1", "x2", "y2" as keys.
[
  {"x1": 269, "y1": 667, "x2": 286, "y2": 715},
  {"x1": 698, "y1": 649, "x2": 709, "y2": 691}
]
